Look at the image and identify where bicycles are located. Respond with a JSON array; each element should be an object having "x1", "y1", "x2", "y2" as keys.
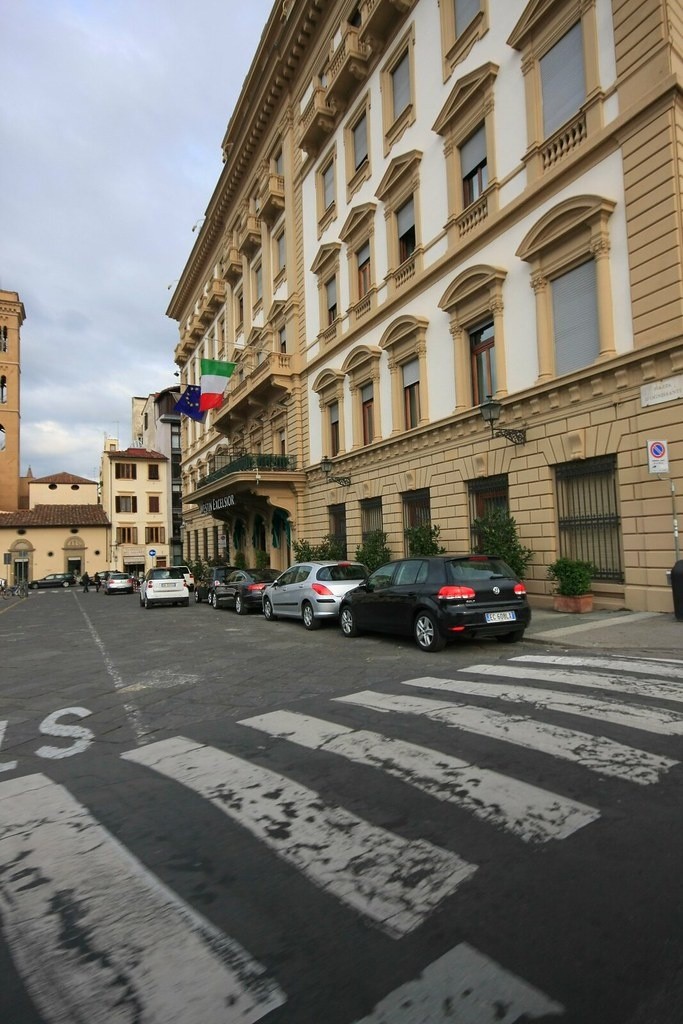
[{"x1": 2, "y1": 582, "x2": 27, "y2": 600}]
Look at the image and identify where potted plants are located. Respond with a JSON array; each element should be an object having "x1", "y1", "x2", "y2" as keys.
[{"x1": 544, "y1": 556, "x2": 594, "y2": 613}]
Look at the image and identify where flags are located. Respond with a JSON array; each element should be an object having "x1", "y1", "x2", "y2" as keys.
[
  {"x1": 174, "y1": 385, "x2": 205, "y2": 420},
  {"x1": 198, "y1": 359, "x2": 237, "y2": 412}
]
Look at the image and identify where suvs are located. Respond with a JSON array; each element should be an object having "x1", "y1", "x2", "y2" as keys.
[{"x1": 0, "y1": 578, "x2": 6, "y2": 591}]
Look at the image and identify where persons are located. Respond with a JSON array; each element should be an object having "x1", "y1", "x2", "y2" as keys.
[{"x1": 81, "y1": 571, "x2": 145, "y2": 593}]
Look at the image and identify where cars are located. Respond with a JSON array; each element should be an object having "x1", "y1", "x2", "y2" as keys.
[
  {"x1": 104, "y1": 573, "x2": 133, "y2": 594},
  {"x1": 138, "y1": 567, "x2": 190, "y2": 609},
  {"x1": 194, "y1": 565, "x2": 242, "y2": 603},
  {"x1": 171, "y1": 565, "x2": 195, "y2": 592},
  {"x1": 262, "y1": 560, "x2": 381, "y2": 630},
  {"x1": 212, "y1": 568, "x2": 285, "y2": 615},
  {"x1": 78, "y1": 570, "x2": 121, "y2": 585},
  {"x1": 339, "y1": 554, "x2": 532, "y2": 653},
  {"x1": 26, "y1": 573, "x2": 77, "y2": 590}
]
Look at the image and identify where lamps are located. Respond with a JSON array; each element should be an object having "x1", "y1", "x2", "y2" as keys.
[
  {"x1": 479, "y1": 394, "x2": 527, "y2": 446},
  {"x1": 320, "y1": 455, "x2": 351, "y2": 487},
  {"x1": 180, "y1": 517, "x2": 193, "y2": 529}
]
